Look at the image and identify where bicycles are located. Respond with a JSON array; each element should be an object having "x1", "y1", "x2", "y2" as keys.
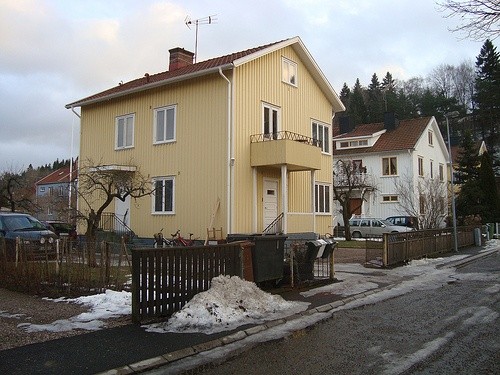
[{"x1": 152, "y1": 227, "x2": 203, "y2": 247}]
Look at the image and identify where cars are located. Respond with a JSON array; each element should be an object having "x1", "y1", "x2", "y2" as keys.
[{"x1": 349, "y1": 218, "x2": 413, "y2": 240}]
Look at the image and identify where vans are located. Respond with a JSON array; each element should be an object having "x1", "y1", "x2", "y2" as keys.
[
  {"x1": 40, "y1": 220, "x2": 78, "y2": 252},
  {"x1": 0, "y1": 212, "x2": 63, "y2": 260},
  {"x1": 382, "y1": 216, "x2": 418, "y2": 231}
]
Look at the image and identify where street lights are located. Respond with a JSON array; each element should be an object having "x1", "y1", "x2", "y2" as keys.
[{"x1": 443, "y1": 106, "x2": 459, "y2": 253}]
